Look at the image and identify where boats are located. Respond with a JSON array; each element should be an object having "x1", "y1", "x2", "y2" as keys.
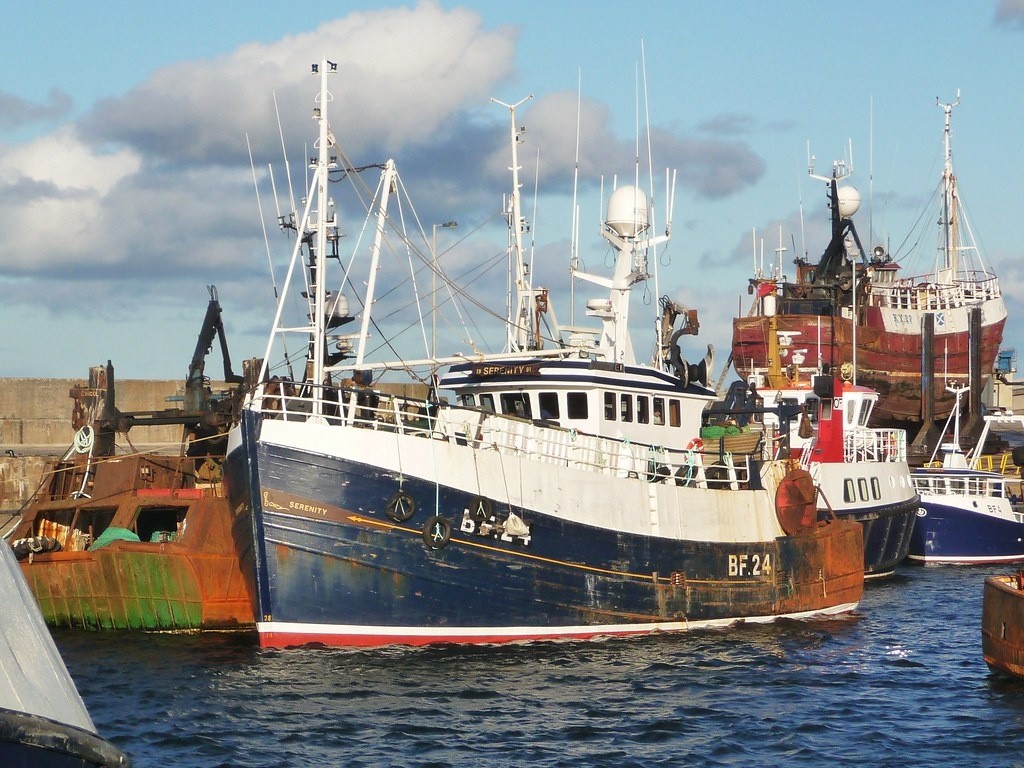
[
  {"x1": 3, "y1": 293, "x2": 258, "y2": 634},
  {"x1": 909, "y1": 383, "x2": 1024, "y2": 566},
  {"x1": 703, "y1": 274, "x2": 918, "y2": 583},
  {"x1": 981, "y1": 573, "x2": 1024, "y2": 680},
  {"x1": 729, "y1": 85, "x2": 1008, "y2": 423},
  {"x1": 220, "y1": 50, "x2": 864, "y2": 653}
]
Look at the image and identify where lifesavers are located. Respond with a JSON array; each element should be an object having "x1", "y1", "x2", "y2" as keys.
[
  {"x1": 705, "y1": 461, "x2": 729, "y2": 489},
  {"x1": 648, "y1": 466, "x2": 671, "y2": 483},
  {"x1": 385, "y1": 491, "x2": 416, "y2": 524},
  {"x1": 884, "y1": 437, "x2": 896, "y2": 459},
  {"x1": 684, "y1": 438, "x2": 703, "y2": 463},
  {"x1": 423, "y1": 515, "x2": 452, "y2": 549},
  {"x1": 468, "y1": 496, "x2": 492, "y2": 522},
  {"x1": 675, "y1": 464, "x2": 698, "y2": 486}
]
